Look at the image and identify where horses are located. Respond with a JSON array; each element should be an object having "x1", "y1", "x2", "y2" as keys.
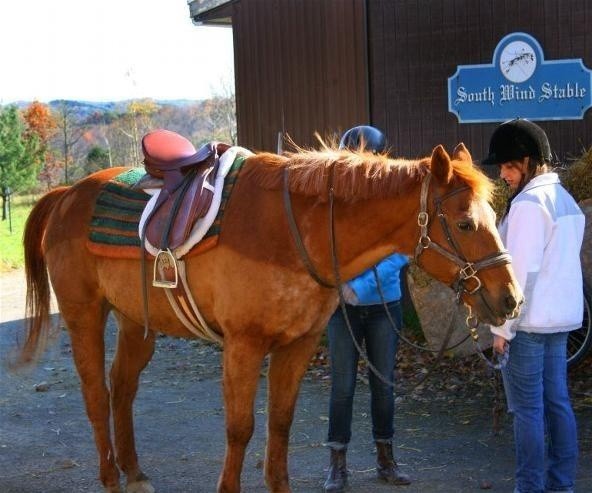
[{"x1": 8, "y1": 129, "x2": 525, "y2": 493}]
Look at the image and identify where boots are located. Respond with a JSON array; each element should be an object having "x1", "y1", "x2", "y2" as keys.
[
  {"x1": 323, "y1": 443, "x2": 347, "y2": 493},
  {"x1": 375, "y1": 440, "x2": 411, "y2": 484}
]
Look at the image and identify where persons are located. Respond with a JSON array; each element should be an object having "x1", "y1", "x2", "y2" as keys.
[
  {"x1": 323, "y1": 123, "x2": 413, "y2": 492},
  {"x1": 483, "y1": 114, "x2": 587, "y2": 492}
]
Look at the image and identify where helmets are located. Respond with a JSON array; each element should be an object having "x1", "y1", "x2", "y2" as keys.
[
  {"x1": 339, "y1": 125, "x2": 386, "y2": 155},
  {"x1": 481, "y1": 117, "x2": 552, "y2": 165}
]
